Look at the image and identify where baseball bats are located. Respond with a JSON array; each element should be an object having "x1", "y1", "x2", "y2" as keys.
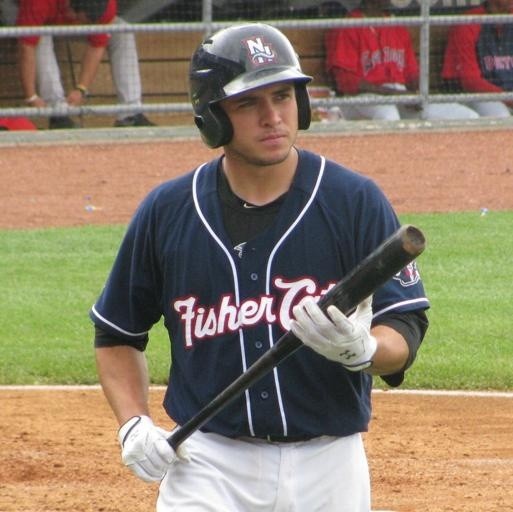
[{"x1": 167, "y1": 224, "x2": 425, "y2": 450}]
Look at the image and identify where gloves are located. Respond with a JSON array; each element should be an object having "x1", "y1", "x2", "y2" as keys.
[
  {"x1": 289, "y1": 291, "x2": 377, "y2": 373},
  {"x1": 117, "y1": 415, "x2": 190, "y2": 485}
]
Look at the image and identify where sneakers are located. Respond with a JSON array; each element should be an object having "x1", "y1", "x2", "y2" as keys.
[{"x1": 46, "y1": 115, "x2": 159, "y2": 127}]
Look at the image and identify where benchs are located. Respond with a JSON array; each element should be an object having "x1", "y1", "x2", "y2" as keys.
[{"x1": 0, "y1": 28, "x2": 462, "y2": 130}]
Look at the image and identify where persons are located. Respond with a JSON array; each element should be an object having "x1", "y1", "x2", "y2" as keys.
[
  {"x1": 17, "y1": 0, "x2": 157, "y2": 131},
  {"x1": 442, "y1": 0, "x2": 513, "y2": 118},
  {"x1": 88, "y1": 23, "x2": 432, "y2": 512},
  {"x1": 323, "y1": 0, "x2": 479, "y2": 122}
]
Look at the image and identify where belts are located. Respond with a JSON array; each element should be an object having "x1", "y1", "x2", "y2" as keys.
[{"x1": 198, "y1": 427, "x2": 318, "y2": 444}]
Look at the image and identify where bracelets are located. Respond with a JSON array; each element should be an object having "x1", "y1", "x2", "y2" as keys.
[
  {"x1": 24, "y1": 94, "x2": 38, "y2": 104},
  {"x1": 75, "y1": 82, "x2": 87, "y2": 96}
]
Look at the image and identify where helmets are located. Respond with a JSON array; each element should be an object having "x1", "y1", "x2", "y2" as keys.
[{"x1": 190, "y1": 23, "x2": 312, "y2": 151}]
